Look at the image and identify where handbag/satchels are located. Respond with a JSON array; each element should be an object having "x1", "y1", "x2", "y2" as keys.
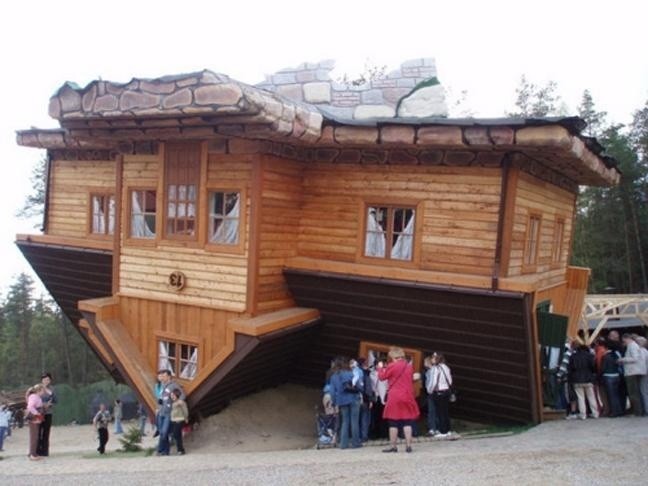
[
  {"x1": 342, "y1": 382, "x2": 359, "y2": 393},
  {"x1": 384, "y1": 390, "x2": 388, "y2": 402}
]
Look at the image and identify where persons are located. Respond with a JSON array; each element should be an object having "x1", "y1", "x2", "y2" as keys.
[
  {"x1": 113, "y1": 399, "x2": 123, "y2": 434},
  {"x1": 323, "y1": 346, "x2": 462, "y2": 452},
  {"x1": 541, "y1": 329, "x2": 648, "y2": 420},
  {"x1": 135, "y1": 400, "x2": 151, "y2": 435},
  {"x1": 93, "y1": 403, "x2": 112, "y2": 453},
  {"x1": 0, "y1": 373, "x2": 58, "y2": 459},
  {"x1": 154, "y1": 370, "x2": 190, "y2": 455}
]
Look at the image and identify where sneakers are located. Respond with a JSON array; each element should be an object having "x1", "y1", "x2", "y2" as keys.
[
  {"x1": 566, "y1": 408, "x2": 647, "y2": 420},
  {"x1": 320, "y1": 431, "x2": 461, "y2": 453},
  {"x1": 28, "y1": 453, "x2": 47, "y2": 461},
  {"x1": 155, "y1": 449, "x2": 188, "y2": 456}
]
